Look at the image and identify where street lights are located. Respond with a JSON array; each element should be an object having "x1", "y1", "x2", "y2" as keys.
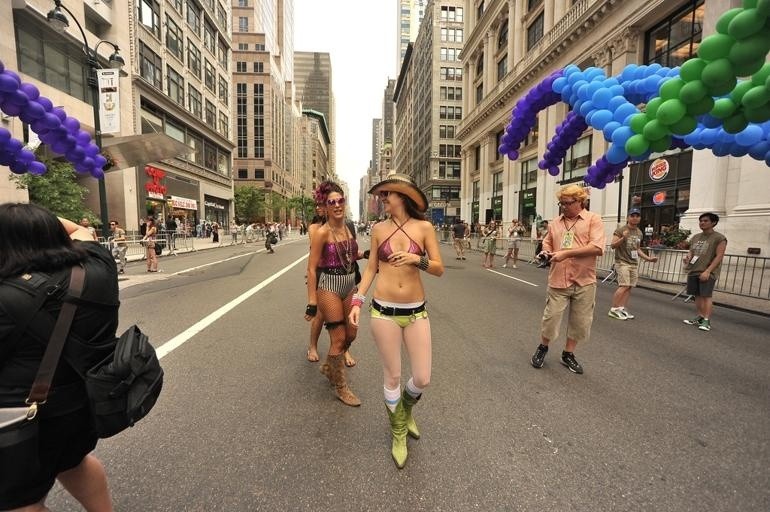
[{"x1": 46, "y1": 0, "x2": 125, "y2": 252}]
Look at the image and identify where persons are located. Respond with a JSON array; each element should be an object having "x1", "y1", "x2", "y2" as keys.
[
  {"x1": 303, "y1": 180, "x2": 362, "y2": 407},
  {"x1": 642, "y1": 224, "x2": 680, "y2": 245},
  {"x1": 195, "y1": 220, "x2": 219, "y2": 242},
  {"x1": 264, "y1": 224, "x2": 274, "y2": 253},
  {"x1": 107, "y1": 220, "x2": 127, "y2": 272},
  {"x1": 165, "y1": 215, "x2": 177, "y2": 250},
  {"x1": 346, "y1": 173, "x2": 445, "y2": 469},
  {"x1": 81, "y1": 216, "x2": 98, "y2": 241},
  {"x1": 139, "y1": 215, "x2": 162, "y2": 271},
  {"x1": 607, "y1": 207, "x2": 658, "y2": 320},
  {"x1": 452, "y1": 213, "x2": 551, "y2": 269},
  {"x1": 306, "y1": 185, "x2": 370, "y2": 367},
  {"x1": 1, "y1": 202, "x2": 123, "y2": 512},
  {"x1": 230, "y1": 220, "x2": 286, "y2": 244},
  {"x1": 350, "y1": 220, "x2": 376, "y2": 236},
  {"x1": 529, "y1": 183, "x2": 607, "y2": 372},
  {"x1": 680, "y1": 212, "x2": 728, "y2": 331}
]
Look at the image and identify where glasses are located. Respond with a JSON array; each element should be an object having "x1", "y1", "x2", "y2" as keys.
[
  {"x1": 558, "y1": 200, "x2": 577, "y2": 207},
  {"x1": 379, "y1": 192, "x2": 390, "y2": 199},
  {"x1": 328, "y1": 198, "x2": 345, "y2": 206}
]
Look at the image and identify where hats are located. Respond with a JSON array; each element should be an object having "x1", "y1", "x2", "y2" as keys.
[
  {"x1": 368, "y1": 174, "x2": 428, "y2": 213},
  {"x1": 628, "y1": 208, "x2": 641, "y2": 214}
]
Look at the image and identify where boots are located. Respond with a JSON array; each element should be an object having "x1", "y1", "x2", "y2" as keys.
[
  {"x1": 403, "y1": 385, "x2": 422, "y2": 439},
  {"x1": 319, "y1": 342, "x2": 362, "y2": 406},
  {"x1": 385, "y1": 386, "x2": 408, "y2": 469}
]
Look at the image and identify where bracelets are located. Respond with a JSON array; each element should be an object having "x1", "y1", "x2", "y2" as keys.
[{"x1": 418, "y1": 256, "x2": 428, "y2": 272}]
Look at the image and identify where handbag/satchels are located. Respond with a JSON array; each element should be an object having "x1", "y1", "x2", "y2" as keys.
[
  {"x1": 1, "y1": 400, "x2": 42, "y2": 490},
  {"x1": 85, "y1": 325, "x2": 164, "y2": 438},
  {"x1": 155, "y1": 243, "x2": 161, "y2": 255}
]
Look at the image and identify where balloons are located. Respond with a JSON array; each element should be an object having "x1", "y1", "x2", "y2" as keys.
[
  {"x1": 499, "y1": 1, "x2": 769, "y2": 188},
  {"x1": 0, "y1": 59, "x2": 110, "y2": 181}
]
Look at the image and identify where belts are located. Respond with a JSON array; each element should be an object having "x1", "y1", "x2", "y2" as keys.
[{"x1": 372, "y1": 299, "x2": 425, "y2": 315}]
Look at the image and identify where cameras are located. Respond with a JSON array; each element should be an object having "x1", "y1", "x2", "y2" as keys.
[{"x1": 537, "y1": 251, "x2": 552, "y2": 269}]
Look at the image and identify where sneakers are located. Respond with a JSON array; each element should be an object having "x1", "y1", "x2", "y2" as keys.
[
  {"x1": 531, "y1": 344, "x2": 548, "y2": 368},
  {"x1": 561, "y1": 350, "x2": 583, "y2": 373},
  {"x1": 608, "y1": 307, "x2": 635, "y2": 321},
  {"x1": 456, "y1": 257, "x2": 518, "y2": 269},
  {"x1": 682, "y1": 315, "x2": 711, "y2": 332}
]
